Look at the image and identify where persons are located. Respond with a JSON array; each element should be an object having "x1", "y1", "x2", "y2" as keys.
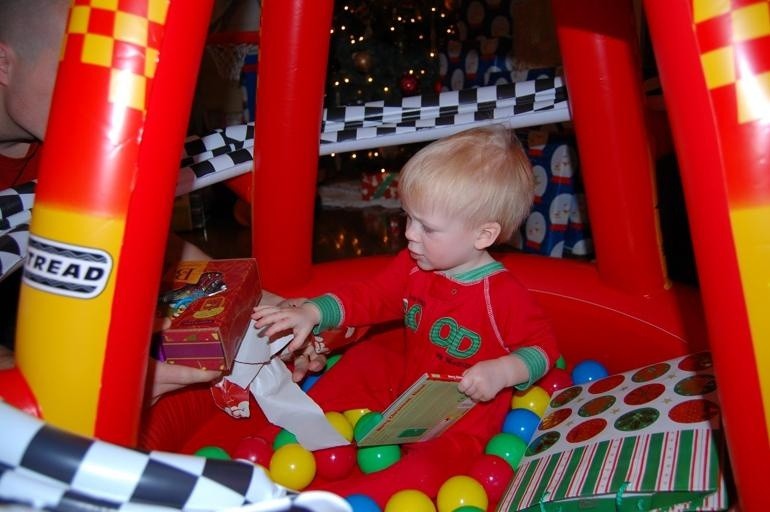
[
  {"x1": 0, "y1": 0, "x2": 328, "y2": 409},
  {"x1": 250, "y1": 122, "x2": 561, "y2": 478}
]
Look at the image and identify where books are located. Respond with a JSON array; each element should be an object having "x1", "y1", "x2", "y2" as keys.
[{"x1": 357, "y1": 372, "x2": 480, "y2": 447}]
